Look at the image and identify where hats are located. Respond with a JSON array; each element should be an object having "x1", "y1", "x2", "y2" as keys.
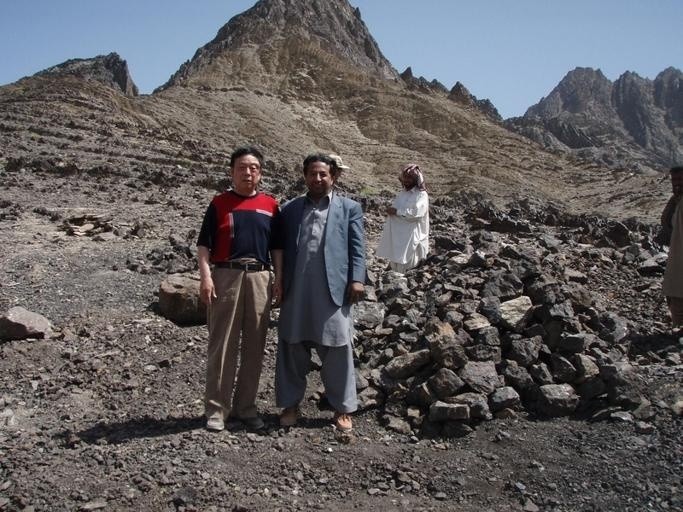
[{"x1": 328, "y1": 154, "x2": 350, "y2": 169}]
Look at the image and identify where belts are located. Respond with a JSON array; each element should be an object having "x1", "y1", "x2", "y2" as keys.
[{"x1": 216, "y1": 262, "x2": 266, "y2": 271}]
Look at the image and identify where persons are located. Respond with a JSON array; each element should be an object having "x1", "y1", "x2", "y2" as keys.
[
  {"x1": 193, "y1": 146, "x2": 281, "y2": 433},
  {"x1": 374, "y1": 164, "x2": 432, "y2": 272},
  {"x1": 653, "y1": 166, "x2": 683, "y2": 328},
  {"x1": 274, "y1": 151, "x2": 368, "y2": 433}
]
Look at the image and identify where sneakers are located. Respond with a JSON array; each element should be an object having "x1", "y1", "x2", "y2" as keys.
[
  {"x1": 279, "y1": 407, "x2": 302, "y2": 427},
  {"x1": 240, "y1": 416, "x2": 265, "y2": 430},
  {"x1": 206, "y1": 416, "x2": 224, "y2": 431},
  {"x1": 333, "y1": 411, "x2": 352, "y2": 431}
]
